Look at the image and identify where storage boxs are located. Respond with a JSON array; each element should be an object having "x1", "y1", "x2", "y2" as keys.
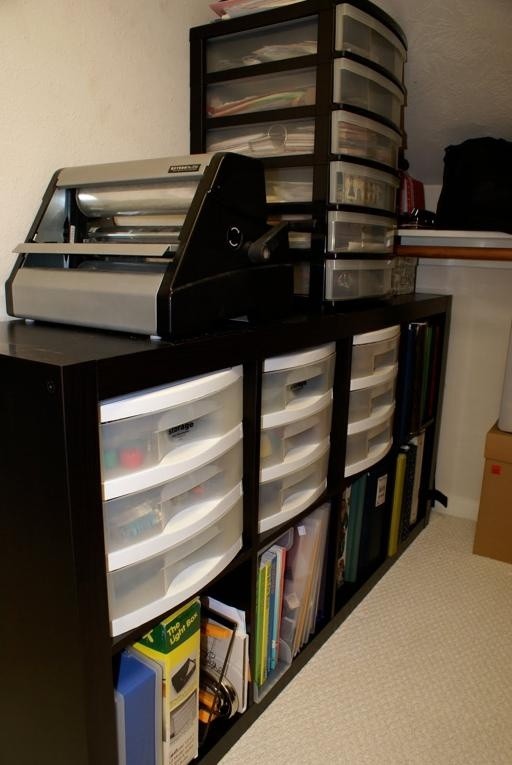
[{"x1": 469, "y1": 420, "x2": 511, "y2": 566}]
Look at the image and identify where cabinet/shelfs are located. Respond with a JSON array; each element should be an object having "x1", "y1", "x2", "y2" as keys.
[
  {"x1": 187, "y1": 2, "x2": 411, "y2": 319},
  {"x1": 1, "y1": 289, "x2": 458, "y2": 765}
]
